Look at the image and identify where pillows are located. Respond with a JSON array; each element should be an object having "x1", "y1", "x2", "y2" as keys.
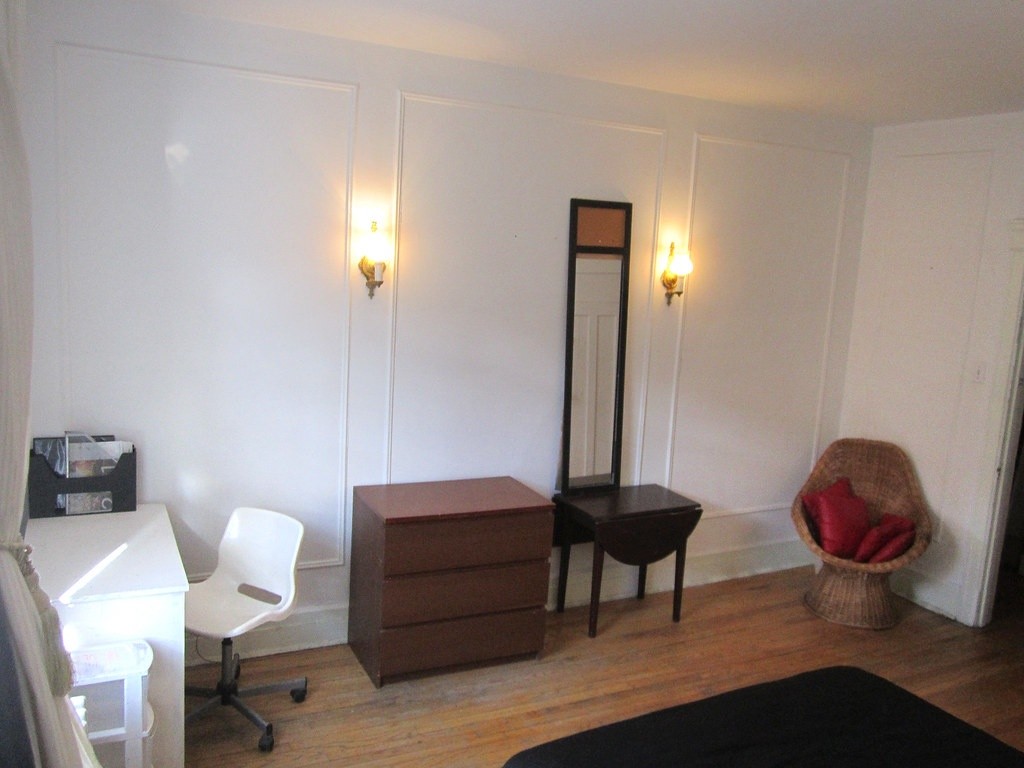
[{"x1": 802, "y1": 477, "x2": 917, "y2": 565}]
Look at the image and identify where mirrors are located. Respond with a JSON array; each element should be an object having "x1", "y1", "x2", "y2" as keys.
[{"x1": 561, "y1": 197, "x2": 634, "y2": 494}]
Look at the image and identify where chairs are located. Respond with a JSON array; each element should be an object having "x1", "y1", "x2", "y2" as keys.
[
  {"x1": 790, "y1": 437, "x2": 932, "y2": 630},
  {"x1": 185, "y1": 506, "x2": 308, "y2": 753}
]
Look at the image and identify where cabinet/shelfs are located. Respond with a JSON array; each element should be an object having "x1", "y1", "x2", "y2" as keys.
[
  {"x1": 347, "y1": 476, "x2": 558, "y2": 690},
  {"x1": 21, "y1": 502, "x2": 190, "y2": 768}
]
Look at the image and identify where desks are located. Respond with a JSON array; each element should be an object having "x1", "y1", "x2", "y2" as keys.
[{"x1": 551, "y1": 483, "x2": 704, "y2": 639}]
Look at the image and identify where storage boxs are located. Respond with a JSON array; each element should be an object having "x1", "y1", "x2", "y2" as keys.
[{"x1": 29, "y1": 432, "x2": 137, "y2": 520}]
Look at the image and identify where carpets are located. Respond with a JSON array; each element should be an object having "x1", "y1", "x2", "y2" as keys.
[{"x1": 500, "y1": 664, "x2": 1024, "y2": 768}]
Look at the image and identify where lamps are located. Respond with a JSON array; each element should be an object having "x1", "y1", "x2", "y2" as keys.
[
  {"x1": 357, "y1": 220, "x2": 389, "y2": 297},
  {"x1": 660, "y1": 241, "x2": 692, "y2": 305}
]
